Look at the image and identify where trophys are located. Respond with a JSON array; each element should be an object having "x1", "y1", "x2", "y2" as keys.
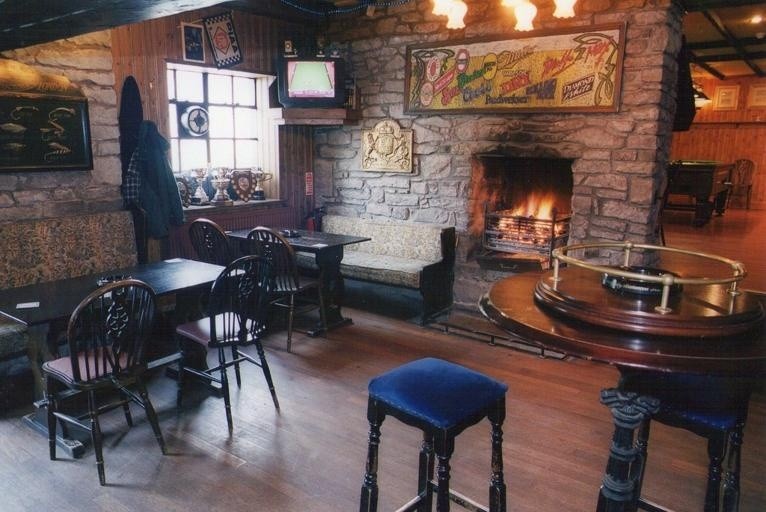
[{"x1": 188, "y1": 161, "x2": 273, "y2": 208}]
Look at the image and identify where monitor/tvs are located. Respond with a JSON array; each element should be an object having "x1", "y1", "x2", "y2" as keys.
[{"x1": 276, "y1": 57, "x2": 346, "y2": 109}]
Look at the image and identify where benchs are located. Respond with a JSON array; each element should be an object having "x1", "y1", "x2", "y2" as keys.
[
  {"x1": 287, "y1": 211, "x2": 457, "y2": 327},
  {"x1": 0, "y1": 209, "x2": 164, "y2": 379}
]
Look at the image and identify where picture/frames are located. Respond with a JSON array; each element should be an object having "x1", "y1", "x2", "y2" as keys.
[
  {"x1": 712, "y1": 83, "x2": 766, "y2": 113},
  {"x1": 180, "y1": 19, "x2": 207, "y2": 66},
  {"x1": 401, "y1": 18, "x2": 631, "y2": 119},
  {"x1": 0, "y1": 90, "x2": 98, "y2": 175}
]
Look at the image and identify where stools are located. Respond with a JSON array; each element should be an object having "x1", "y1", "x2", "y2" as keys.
[
  {"x1": 627, "y1": 390, "x2": 750, "y2": 511},
  {"x1": 358, "y1": 354, "x2": 511, "y2": 510}
]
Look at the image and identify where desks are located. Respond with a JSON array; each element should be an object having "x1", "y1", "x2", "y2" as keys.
[
  {"x1": 217, "y1": 224, "x2": 376, "y2": 339},
  {"x1": 474, "y1": 263, "x2": 765, "y2": 511},
  {"x1": 2, "y1": 256, "x2": 257, "y2": 460},
  {"x1": 661, "y1": 160, "x2": 729, "y2": 228}
]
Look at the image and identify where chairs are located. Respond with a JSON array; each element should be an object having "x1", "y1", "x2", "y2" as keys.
[
  {"x1": 39, "y1": 275, "x2": 169, "y2": 487},
  {"x1": 175, "y1": 255, "x2": 280, "y2": 434},
  {"x1": 659, "y1": 159, "x2": 685, "y2": 248},
  {"x1": 723, "y1": 158, "x2": 756, "y2": 213},
  {"x1": 185, "y1": 217, "x2": 331, "y2": 354}
]
